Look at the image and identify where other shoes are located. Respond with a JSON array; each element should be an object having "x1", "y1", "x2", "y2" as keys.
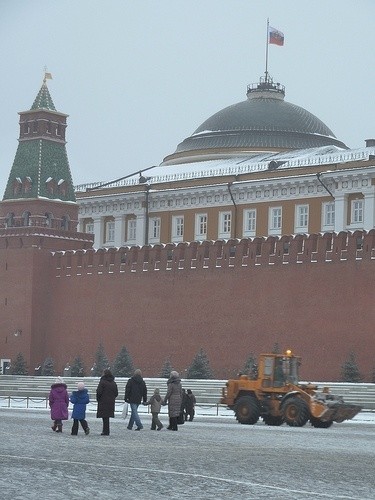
[
  {"x1": 157, "y1": 426, "x2": 163, "y2": 431},
  {"x1": 85, "y1": 428, "x2": 90, "y2": 435},
  {"x1": 71, "y1": 433, "x2": 77, "y2": 435},
  {"x1": 151, "y1": 427, "x2": 156, "y2": 430},
  {"x1": 101, "y1": 432, "x2": 109, "y2": 436},
  {"x1": 172, "y1": 428, "x2": 177, "y2": 431},
  {"x1": 127, "y1": 426, "x2": 132, "y2": 430},
  {"x1": 167, "y1": 427, "x2": 171, "y2": 430},
  {"x1": 135, "y1": 427, "x2": 143, "y2": 431}
]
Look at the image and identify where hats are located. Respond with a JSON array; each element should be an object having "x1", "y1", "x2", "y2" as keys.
[
  {"x1": 103, "y1": 369, "x2": 111, "y2": 376},
  {"x1": 135, "y1": 369, "x2": 142, "y2": 375},
  {"x1": 171, "y1": 370, "x2": 180, "y2": 378},
  {"x1": 77, "y1": 382, "x2": 85, "y2": 390},
  {"x1": 154, "y1": 387, "x2": 160, "y2": 394},
  {"x1": 54, "y1": 374, "x2": 64, "y2": 383}
]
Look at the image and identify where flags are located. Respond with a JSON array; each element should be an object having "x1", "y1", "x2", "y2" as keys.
[{"x1": 268, "y1": 27, "x2": 285, "y2": 46}]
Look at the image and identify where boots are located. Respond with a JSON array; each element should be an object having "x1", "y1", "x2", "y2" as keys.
[
  {"x1": 56, "y1": 424, "x2": 63, "y2": 432},
  {"x1": 52, "y1": 422, "x2": 57, "y2": 431}
]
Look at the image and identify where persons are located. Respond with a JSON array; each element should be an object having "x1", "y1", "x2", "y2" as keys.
[
  {"x1": 124, "y1": 369, "x2": 147, "y2": 432},
  {"x1": 273, "y1": 361, "x2": 286, "y2": 383},
  {"x1": 49, "y1": 376, "x2": 70, "y2": 433},
  {"x1": 164, "y1": 371, "x2": 196, "y2": 431},
  {"x1": 70, "y1": 383, "x2": 90, "y2": 435},
  {"x1": 96, "y1": 370, "x2": 118, "y2": 435},
  {"x1": 144, "y1": 388, "x2": 169, "y2": 431}
]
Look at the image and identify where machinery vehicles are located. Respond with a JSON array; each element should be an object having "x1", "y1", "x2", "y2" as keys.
[{"x1": 220, "y1": 350, "x2": 363, "y2": 428}]
